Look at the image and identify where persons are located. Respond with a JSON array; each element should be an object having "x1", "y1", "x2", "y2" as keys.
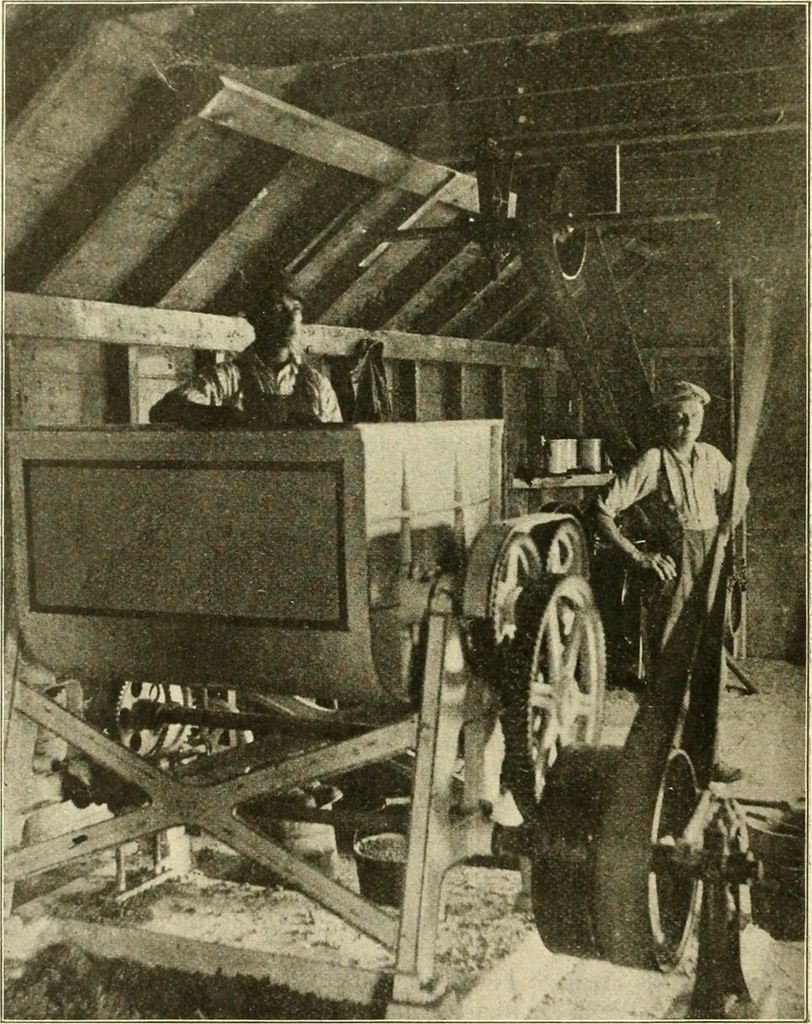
[
  {"x1": 594, "y1": 379, "x2": 751, "y2": 784},
  {"x1": 147, "y1": 265, "x2": 346, "y2": 428}
]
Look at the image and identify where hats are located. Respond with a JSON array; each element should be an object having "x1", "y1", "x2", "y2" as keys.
[{"x1": 652, "y1": 381, "x2": 711, "y2": 407}]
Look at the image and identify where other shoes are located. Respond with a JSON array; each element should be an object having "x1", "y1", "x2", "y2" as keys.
[{"x1": 710, "y1": 760, "x2": 744, "y2": 783}]
